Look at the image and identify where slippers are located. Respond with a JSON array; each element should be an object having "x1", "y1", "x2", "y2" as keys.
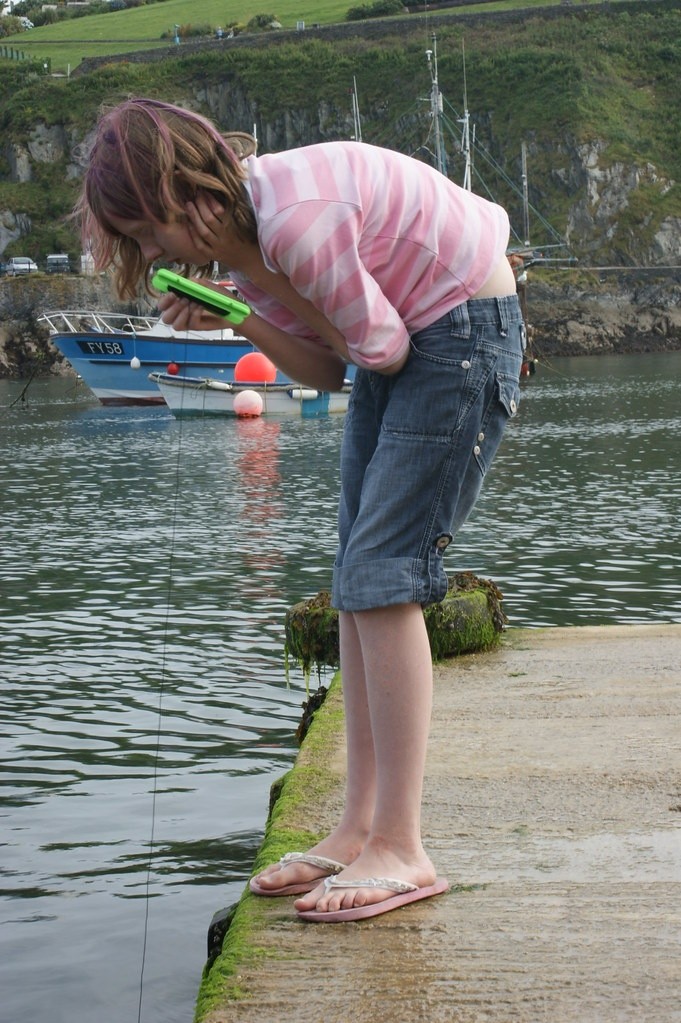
[
  {"x1": 292, "y1": 867, "x2": 449, "y2": 922},
  {"x1": 247, "y1": 851, "x2": 350, "y2": 896}
]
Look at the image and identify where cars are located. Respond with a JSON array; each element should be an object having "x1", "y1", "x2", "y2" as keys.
[
  {"x1": 5, "y1": 256, "x2": 38, "y2": 277},
  {"x1": 42, "y1": 254, "x2": 72, "y2": 275},
  {"x1": 0, "y1": 261, "x2": 7, "y2": 277}
]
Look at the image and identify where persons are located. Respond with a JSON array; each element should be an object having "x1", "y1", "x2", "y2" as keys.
[{"x1": 67, "y1": 98, "x2": 526, "y2": 921}]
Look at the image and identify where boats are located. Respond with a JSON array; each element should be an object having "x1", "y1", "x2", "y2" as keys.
[
  {"x1": 148, "y1": 370, "x2": 357, "y2": 419},
  {"x1": 37, "y1": 25, "x2": 583, "y2": 408}
]
